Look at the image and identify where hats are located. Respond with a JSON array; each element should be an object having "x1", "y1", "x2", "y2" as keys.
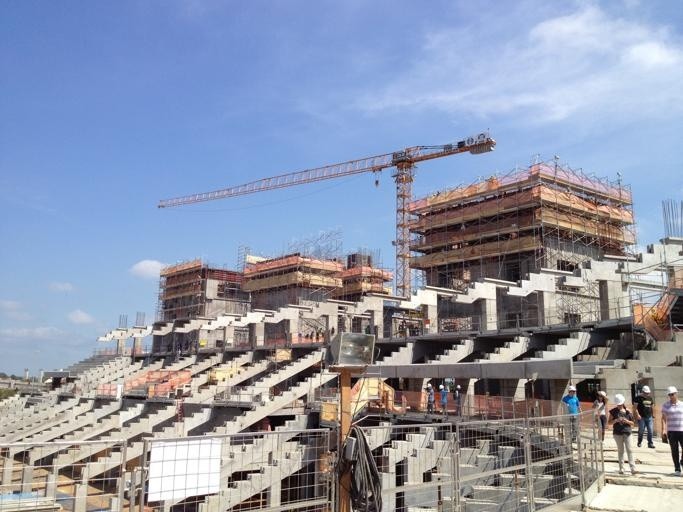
[{"x1": 597, "y1": 390, "x2": 606, "y2": 397}]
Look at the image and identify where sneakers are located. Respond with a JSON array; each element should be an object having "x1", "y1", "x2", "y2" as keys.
[
  {"x1": 638, "y1": 443, "x2": 655, "y2": 448},
  {"x1": 673, "y1": 470, "x2": 681, "y2": 476},
  {"x1": 618, "y1": 468, "x2": 639, "y2": 474}
]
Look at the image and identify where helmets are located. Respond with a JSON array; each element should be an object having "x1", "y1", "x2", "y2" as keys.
[
  {"x1": 667, "y1": 386, "x2": 678, "y2": 396},
  {"x1": 427, "y1": 384, "x2": 461, "y2": 389},
  {"x1": 614, "y1": 394, "x2": 626, "y2": 406},
  {"x1": 568, "y1": 386, "x2": 576, "y2": 391},
  {"x1": 642, "y1": 385, "x2": 651, "y2": 393}
]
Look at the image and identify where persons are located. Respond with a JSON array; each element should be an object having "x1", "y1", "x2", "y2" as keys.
[
  {"x1": 634, "y1": 385, "x2": 656, "y2": 448},
  {"x1": 591, "y1": 390, "x2": 608, "y2": 441},
  {"x1": 608, "y1": 393, "x2": 639, "y2": 474},
  {"x1": 424, "y1": 383, "x2": 435, "y2": 414},
  {"x1": 439, "y1": 384, "x2": 448, "y2": 415},
  {"x1": 560, "y1": 386, "x2": 584, "y2": 443},
  {"x1": 453, "y1": 384, "x2": 463, "y2": 416},
  {"x1": 660, "y1": 386, "x2": 683, "y2": 476}
]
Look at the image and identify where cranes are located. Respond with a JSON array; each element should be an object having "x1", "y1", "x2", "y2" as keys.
[{"x1": 157, "y1": 130, "x2": 497, "y2": 298}]
